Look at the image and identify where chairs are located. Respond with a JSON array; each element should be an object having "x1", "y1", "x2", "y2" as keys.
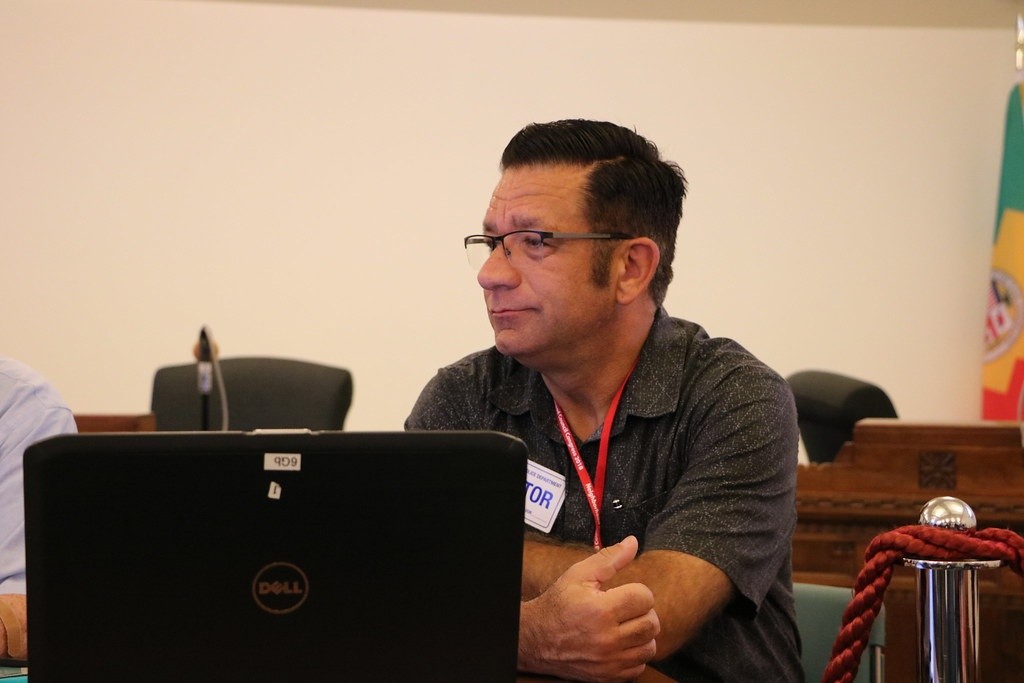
[
  {"x1": 153, "y1": 356, "x2": 353, "y2": 432},
  {"x1": 794, "y1": 582, "x2": 887, "y2": 683}
]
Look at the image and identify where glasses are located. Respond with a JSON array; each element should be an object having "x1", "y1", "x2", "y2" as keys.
[{"x1": 465, "y1": 229, "x2": 633, "y2": 268}]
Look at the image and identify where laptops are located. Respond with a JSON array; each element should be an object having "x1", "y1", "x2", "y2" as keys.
[{"x1": 23, "y1": 429, "x2": 528, "y2": 683}]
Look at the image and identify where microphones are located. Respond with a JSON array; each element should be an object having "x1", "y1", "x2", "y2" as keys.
[{"x1": 193, "y1": 329, "x2": 219, "y2": 363}]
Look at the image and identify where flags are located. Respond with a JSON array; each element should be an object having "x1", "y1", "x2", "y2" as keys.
[{"x1": 980, "y1": 84, "x2": 1024, "y2": 423}]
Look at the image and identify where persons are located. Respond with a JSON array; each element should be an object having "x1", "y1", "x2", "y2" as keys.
[
  {"x1": 0, "y1": 358, "x2": 79, "y2": 666},
  {"x1": 404, "y1": 119, "x2": 808, "y2": 683}
]
